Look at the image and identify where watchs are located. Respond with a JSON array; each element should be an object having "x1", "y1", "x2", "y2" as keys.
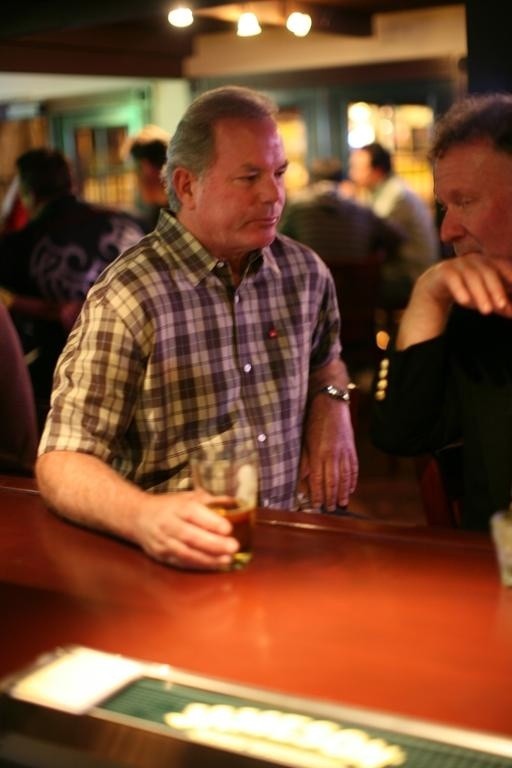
[{"x1": 318, "y1": 385, "x2": 350, "y2": 403}]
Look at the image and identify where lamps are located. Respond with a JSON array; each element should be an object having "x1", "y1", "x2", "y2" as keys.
[
  {"x1": 284, "y1": 11, "x2": 313, "y2": 38},
  {"x1": 167, "y1": 7, "x2": 194, "y2": 29},
  {"x1": 235, "y1": 12, "x2": 263, "y2": 39}
]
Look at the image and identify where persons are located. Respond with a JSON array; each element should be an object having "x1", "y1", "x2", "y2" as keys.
[
  {"x1": 360, "y1": 93, "x2": 511, "y2": 538},
  {"x1": 0, "y1": 147, "x2": 156, "y2": 401},
  {"x1": 124, "y1": 126, "x2": 170, "y2": 234},
  {"x1": 33, "y1": 87, "x2": 359, "y2": 570},
  {"x1": 283, "y1": 145, "x2": 437, "y2": 285}
]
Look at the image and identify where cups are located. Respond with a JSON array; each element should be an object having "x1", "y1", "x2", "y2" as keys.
[{"x1": 189, "y1": 437, "x2": 257, "y2": 572}]
